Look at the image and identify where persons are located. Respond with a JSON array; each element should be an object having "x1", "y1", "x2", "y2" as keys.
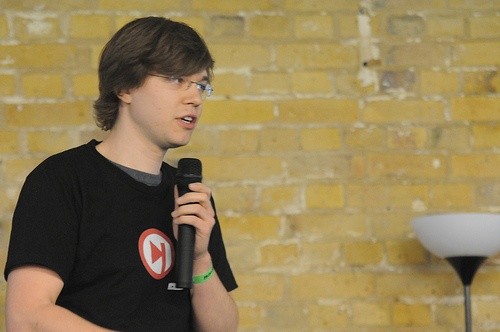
[{"x1": 2, "y1": 15, "x2": 239, "y2": 332}]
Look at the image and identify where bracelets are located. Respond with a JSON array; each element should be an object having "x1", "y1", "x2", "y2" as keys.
[{"x1": 192, "y1": 266, "x2": 215, "y2": 285}]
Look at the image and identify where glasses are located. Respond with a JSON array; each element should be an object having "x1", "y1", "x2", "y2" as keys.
[{"x1": 146, "y1": 73, "x2": 214, "y2": 97}]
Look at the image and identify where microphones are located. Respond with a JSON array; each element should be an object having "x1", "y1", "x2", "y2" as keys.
[{"x1": 174, "y1": 158, "x2": 202, "y2": 289}]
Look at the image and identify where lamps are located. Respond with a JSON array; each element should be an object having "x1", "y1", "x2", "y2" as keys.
[{"x1": 409, "y1": 213, "x2": 500, "y2": 332}]
